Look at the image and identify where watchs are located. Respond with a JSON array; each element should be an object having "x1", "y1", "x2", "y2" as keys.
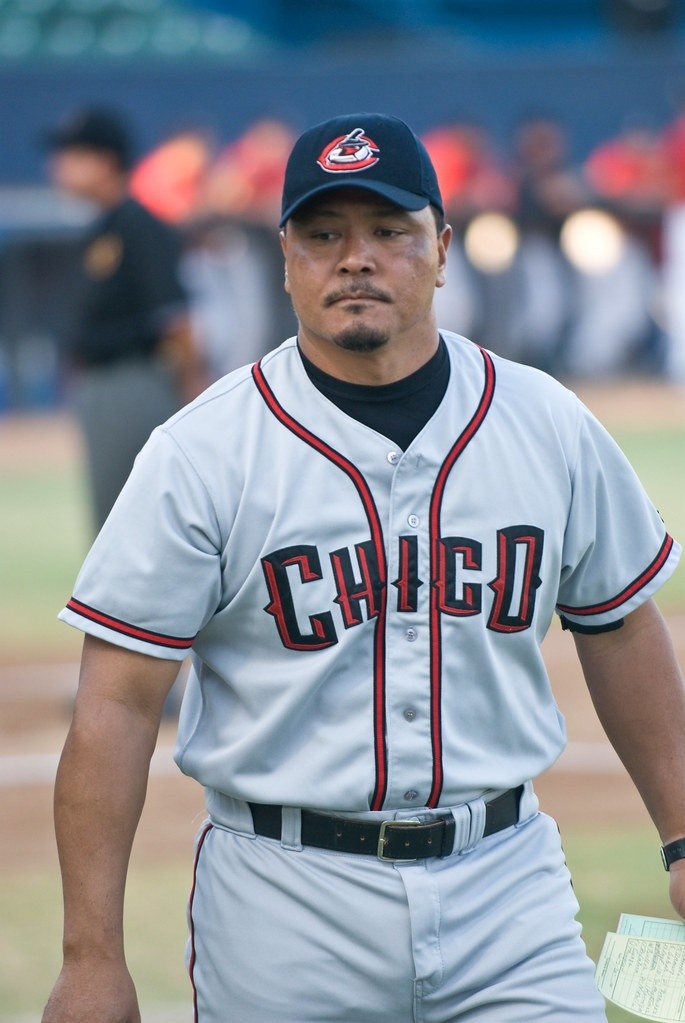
[{"x1": 659, "y1": 836, "x2": 685, "y2": 871}]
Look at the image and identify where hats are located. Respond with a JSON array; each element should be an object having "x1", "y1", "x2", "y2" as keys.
[
  {"x1": 38, "y1": 103, "x2": 147, "y2": 170},
  {"x1": 278, "y1": 112, "x2": 443, "y2": 228}
]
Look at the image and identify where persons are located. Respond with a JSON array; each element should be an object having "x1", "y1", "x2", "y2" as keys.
[{"x1": 39, "y1": 113, "x2": 685, "y2": 1022}]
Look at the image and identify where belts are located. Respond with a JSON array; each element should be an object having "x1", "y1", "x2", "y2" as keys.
[{"x1": 246, "y1": 790, "x2": 522, "y2": 859}]
